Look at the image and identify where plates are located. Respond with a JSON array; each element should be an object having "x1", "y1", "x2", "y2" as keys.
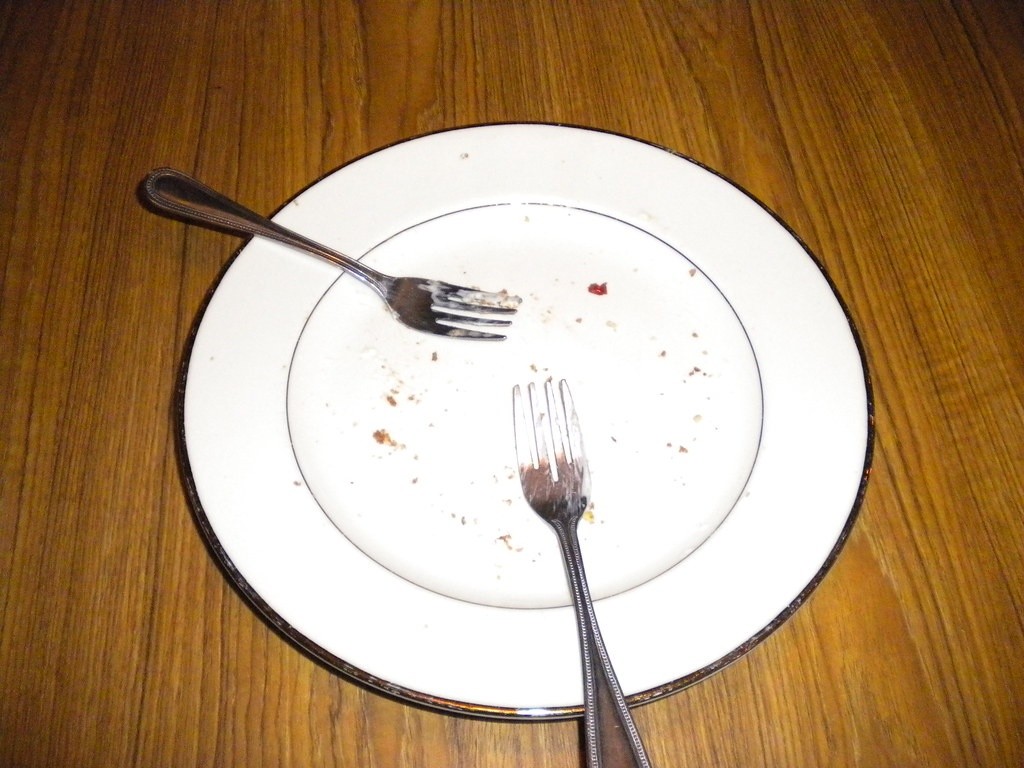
[{"x1": 172, "y1": 122, "x2": 876, "y2": 723}]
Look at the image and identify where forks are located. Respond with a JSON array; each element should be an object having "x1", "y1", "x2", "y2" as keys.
[
  {"x1": 513, "y1": 380, "x2": 653, "y2": 768},
  {"x1": 141, "y1": 166, "x2": 523, "y2": 340}
]
